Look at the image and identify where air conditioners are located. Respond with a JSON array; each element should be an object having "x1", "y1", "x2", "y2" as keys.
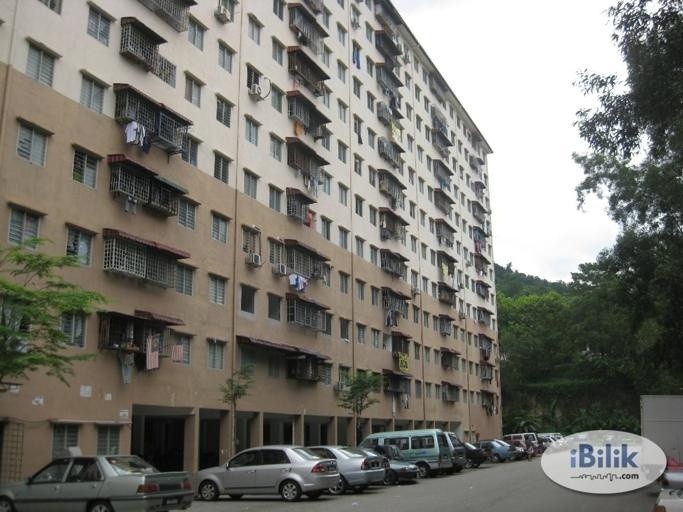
[
  {"x1": 250, "y1": 84, "x2": 262, "y2": 99},
  {"x1": 245, "y1": 251, "x2": 287, "y2": 277},
  {"x1": 217, "y1": 5, "x2": 231, "y2": 24}
]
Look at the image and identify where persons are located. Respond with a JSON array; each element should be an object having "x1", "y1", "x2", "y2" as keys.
[{"x1": 526, "y1": 434, "x2": 536, "y2": 461}]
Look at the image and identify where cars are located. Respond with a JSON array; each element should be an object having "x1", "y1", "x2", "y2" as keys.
[
  {"x1": 654, "y1": 457, "x2": 682, "y2": 511},
  {"x1": 0, "y1": 452, "x2": 194, "y2": 512}
]
